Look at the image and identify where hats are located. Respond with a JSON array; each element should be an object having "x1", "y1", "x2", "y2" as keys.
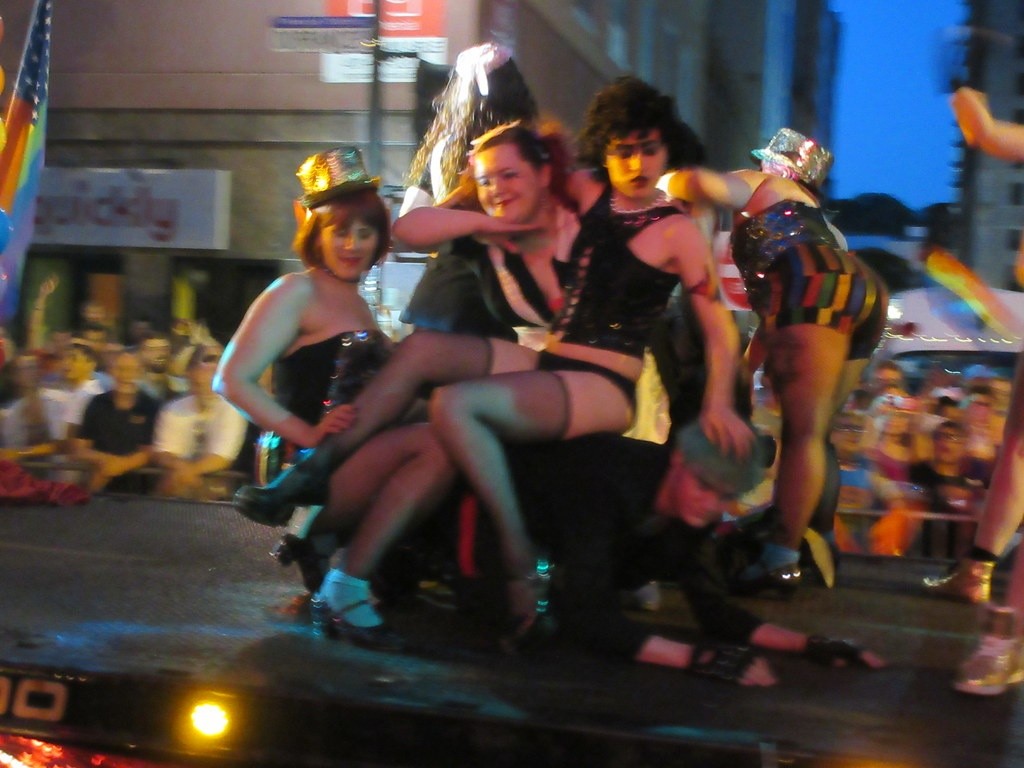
[
  {"x1": 295, "y1": 145, "x2": 380, "y2": 208},
  {"x1": 747, "y1": 127, "x2": 833, "y2": 196}
]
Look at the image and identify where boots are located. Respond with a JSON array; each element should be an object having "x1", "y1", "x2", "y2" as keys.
[{"x1": 922, "y1": 555, "x2": 995, "y2": 604}]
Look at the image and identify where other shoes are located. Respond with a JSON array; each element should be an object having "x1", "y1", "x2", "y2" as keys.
[
  {"x1": 951, "y1": 608, "x2": 1024, "y2": 696},
  {"x1": 377, "y1": 544, "x2": 473, "y2": 617},
  {"x1": 308, "y1": 595, "x2": 410, "y2": 651}
]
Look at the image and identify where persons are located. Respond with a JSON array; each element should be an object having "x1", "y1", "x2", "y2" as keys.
[
  {"x1": 213, "y1": 42, "x2": 886, "y2": 687},
  {"x1": 748, "y1": 362, "x2": 1010, "y2": 563},
  {"x1": 657, "y1": 125, "x2": 891, "y2": 590},
  {"x1": 922, "y1": 85, "x2": 1024, "y2": 694},
  {"x1": 0, "y1": 269, "x2": 247, "y2": 502}
]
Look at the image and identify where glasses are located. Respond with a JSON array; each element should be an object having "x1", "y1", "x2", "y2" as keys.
[
  {"x1": 933, "y1": 431, "x2": 962, "y2": 442},
  {"x1": 875, "y1": 376, "x2": 900, "y2": 393},
  {"x1": 833, "y1": 422, "x2": 867, "y2": 434}
]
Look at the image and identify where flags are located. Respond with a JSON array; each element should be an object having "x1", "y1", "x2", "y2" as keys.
[{"x1": 0, "y1": 0, "x2": 50, "y2": 321}]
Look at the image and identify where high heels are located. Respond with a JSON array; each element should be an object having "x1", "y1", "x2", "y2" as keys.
[
  {"x1": 231, "y1": 463, "x2": 331, "y2": 567},
  {"x1": 718, "y1": 551, "x2": 803, "y2": 602},
  {"x1": 268, "y1": 533, "x2": 323, "y2": 592},
  {"x1": 492, "y1": 546, "x2": 574, "y2": 652}
]
